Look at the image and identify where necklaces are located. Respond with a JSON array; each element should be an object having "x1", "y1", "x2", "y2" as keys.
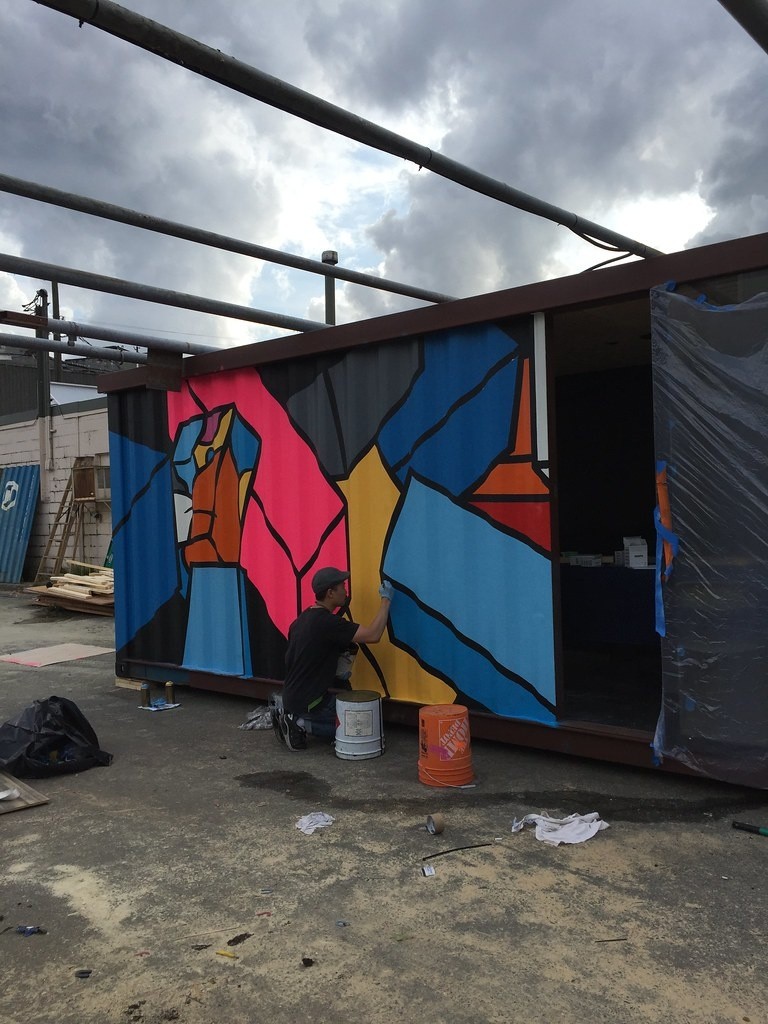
[{"x1": 315, "y1": 602, "x2": 332, "y2": 613}]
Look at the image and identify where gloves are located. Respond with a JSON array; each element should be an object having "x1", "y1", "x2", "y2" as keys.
[
  {"x1": 379, "y1": 579, "x2": 394, "y2": 602},
  {"x1": 340, "y1": 671, "x2": 352, "y2": 681}
]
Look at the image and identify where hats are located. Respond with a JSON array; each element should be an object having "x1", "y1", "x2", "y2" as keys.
[{"x1": 312, "y1": 566, "x2": 350, "y2": 594}]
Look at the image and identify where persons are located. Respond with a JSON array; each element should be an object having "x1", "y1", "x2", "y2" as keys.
[{"x1": 267, "y1": 566, "x2": 396, "y2": 753}]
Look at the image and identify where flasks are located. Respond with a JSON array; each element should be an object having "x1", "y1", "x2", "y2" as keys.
[
  {"x1": 165, "y1": 681, "x2": 175, "y2": 704},
  {"x1": 141, "y1": 683, "x2": 151, "y2": 707}
]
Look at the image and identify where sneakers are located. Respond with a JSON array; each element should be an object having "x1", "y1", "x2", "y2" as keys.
[
  {"x1": 270, "y1": 707, "x2": 286, "y2": 744},
  {"x1": 278, "y1": 714, "x2": 307, "y2": 752}
]
